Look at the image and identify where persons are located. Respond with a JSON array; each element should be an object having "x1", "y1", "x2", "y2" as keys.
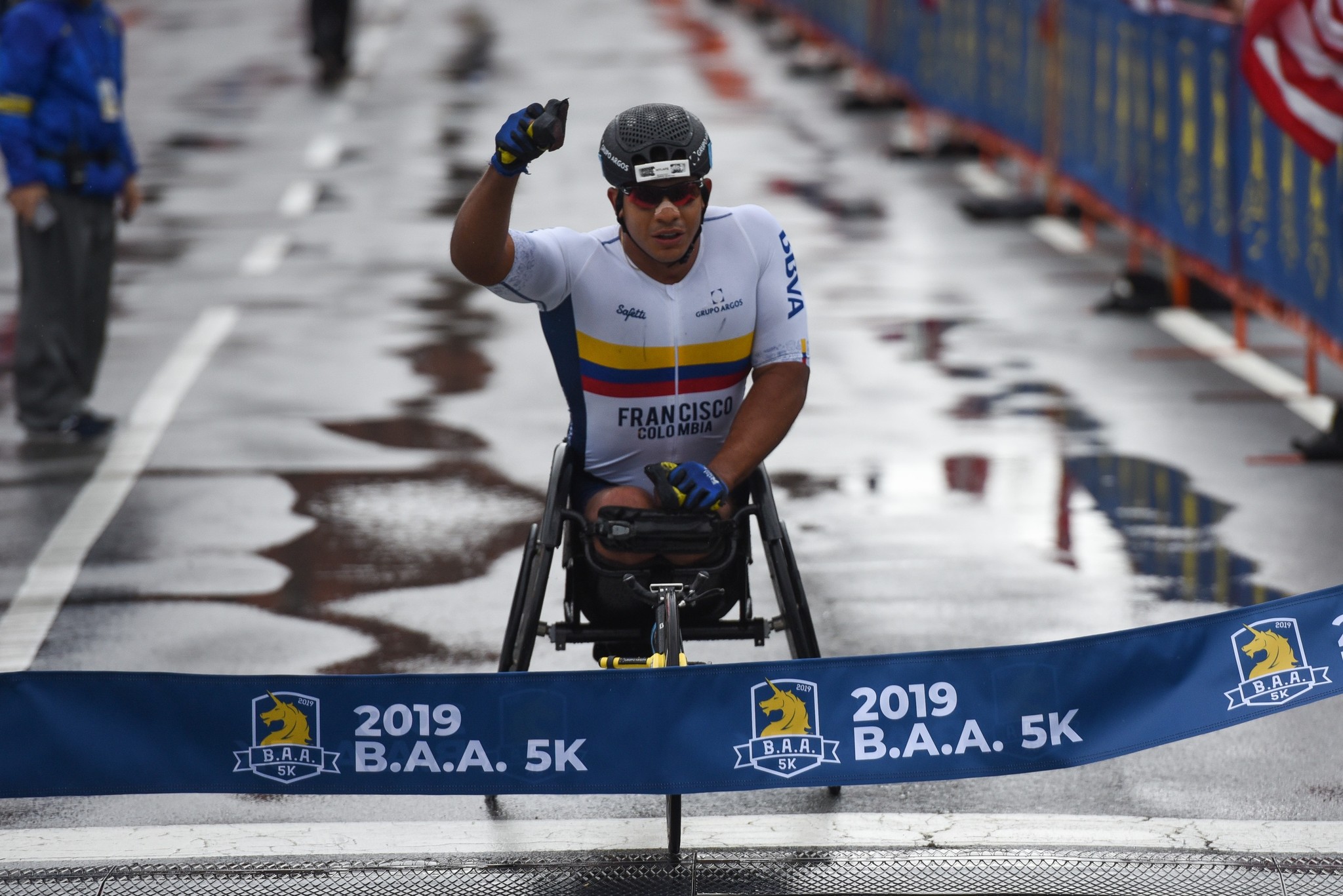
[
  {"x1": 308, "y1": 0, "x2": 355, "y2": 77},
  {"x1": 0, "y1": 0, "x2": 141, "y2": 436},
  {"x1": 449, "y1": 97, "x2": 810, "y2": 570}
]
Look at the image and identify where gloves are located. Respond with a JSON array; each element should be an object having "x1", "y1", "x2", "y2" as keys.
[
  {"x1": 489, "y1": 98, "x2": 569, "y2": 176},
  {"x1": 654, "y1": 461, "x2": 728, "y2": 512}
]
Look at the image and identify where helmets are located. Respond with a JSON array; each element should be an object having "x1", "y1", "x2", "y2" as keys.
[{"x1": 599, "y1": 103, "x2": 713, "y2": 188}]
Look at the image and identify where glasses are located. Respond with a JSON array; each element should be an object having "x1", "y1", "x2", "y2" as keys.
[{"x1": 621, "y1": 180, "x2": 704, "y2": 208}]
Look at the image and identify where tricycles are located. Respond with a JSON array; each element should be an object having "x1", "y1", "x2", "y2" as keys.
[{"x1": 486, "y1": 421, "x2": 841, "y2": 856}]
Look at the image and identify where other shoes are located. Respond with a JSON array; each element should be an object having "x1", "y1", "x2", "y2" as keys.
[{"x1": 25, "y1": 404, "x2": 119, "y2": 447}]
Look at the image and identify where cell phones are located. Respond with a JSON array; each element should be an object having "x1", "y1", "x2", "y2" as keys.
[{"x1": 32, "y1": 202, "x2": 59, "y2": 232}]
